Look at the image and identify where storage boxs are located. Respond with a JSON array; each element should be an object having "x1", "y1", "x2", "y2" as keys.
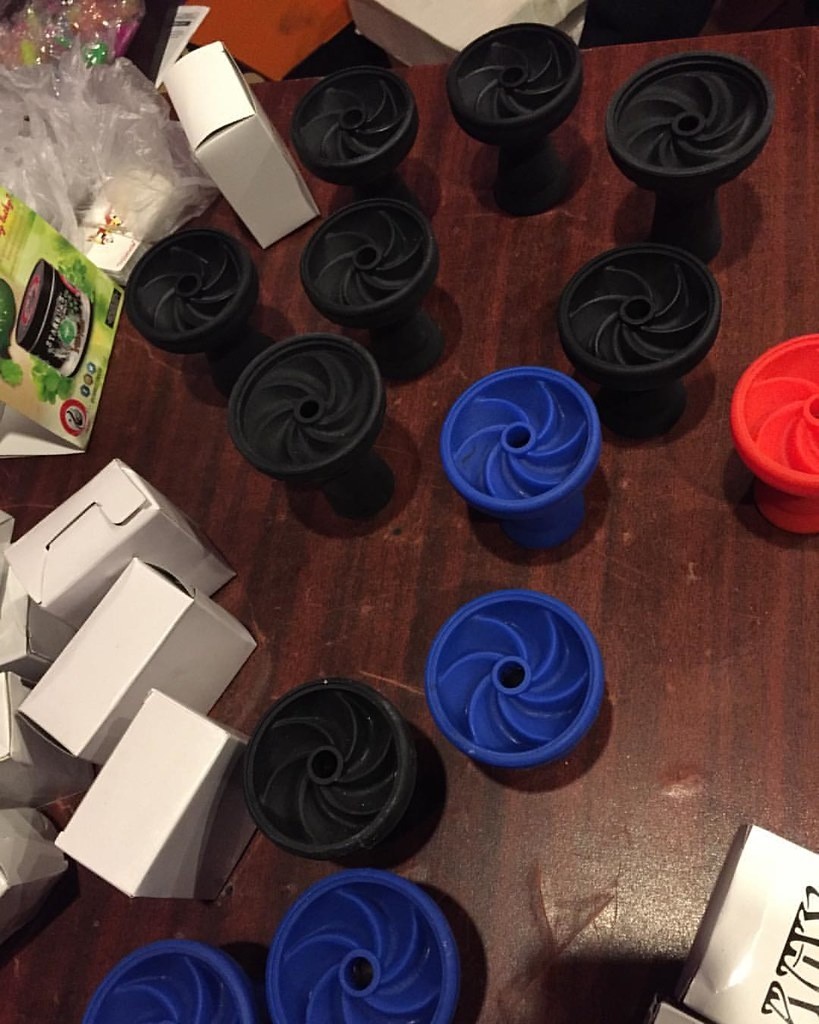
[
  {"x1": 164, "y1": 40, "x2": 321, "y2": 249},
  {"x1": 0, "y1": 454, "x2": 257, "y2": 941},
  {"x1": 642, "y1": 992, "x2": 706, "y2": 1024},
  {"x1": 348, "y1": 0, "x2": 587, "y2": 65},
  {"x1": 676, "y1": 822, "x2": 819, "y2": 1024}
]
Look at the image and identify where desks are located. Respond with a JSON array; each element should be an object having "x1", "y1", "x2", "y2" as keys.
[{"x1": 0, "y1": 24, "x2": 819, "y2": 1024}]
